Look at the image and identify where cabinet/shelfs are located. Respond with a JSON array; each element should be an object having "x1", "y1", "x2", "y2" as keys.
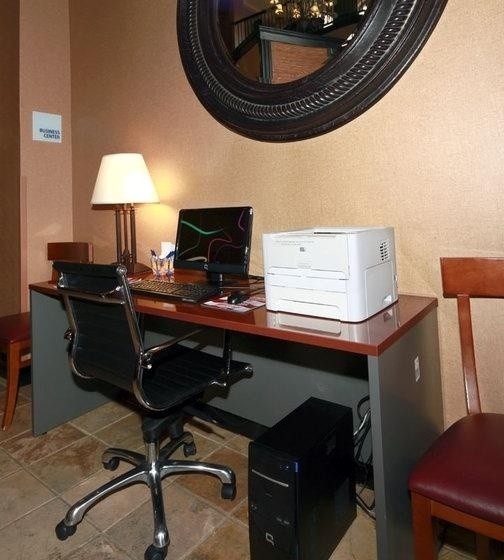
[{"x1": 226, "y1": 25, "x2": 345, "y2": 87}]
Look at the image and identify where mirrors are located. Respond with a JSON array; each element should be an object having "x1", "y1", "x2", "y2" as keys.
[{"x1": 170, "y1": 0, "x2": 450, "y2": 142}]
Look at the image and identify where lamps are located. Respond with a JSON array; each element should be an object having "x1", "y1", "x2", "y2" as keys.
[
  {"x1": 268, "y1": 1, "x2": 368, "y2": 27},
  {"x1": 88, "y1": 153, "x2": 161, "y2": 276}
]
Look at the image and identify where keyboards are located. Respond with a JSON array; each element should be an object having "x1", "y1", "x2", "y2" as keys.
[{"x1": 128, "y1": 279, "x2": 220, "y2": 303}]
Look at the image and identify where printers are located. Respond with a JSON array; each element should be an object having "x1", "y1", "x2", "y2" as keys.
[{"x1": 263, "y1": 227, "x2": 399, "y2": 324}]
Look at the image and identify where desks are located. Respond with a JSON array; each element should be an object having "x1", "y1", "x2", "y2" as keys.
[{"x1": 29, "y1": 266, "x2": 447, "y2": 558}]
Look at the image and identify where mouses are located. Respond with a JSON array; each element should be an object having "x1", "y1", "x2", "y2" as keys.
[{"x1": 228, "y1": 293, "x2": 250, "y2": 304}]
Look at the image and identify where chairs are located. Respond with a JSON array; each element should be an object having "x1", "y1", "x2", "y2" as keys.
[
  {"x1": 51, "y1": 257, "x2": 252, "y2": 559},
  {"x1": 1, "y1": 242, "x2": 92, "y2": 430},
  {"x1": 412, "y1": 256, "x2": 503, "y2": 559}
]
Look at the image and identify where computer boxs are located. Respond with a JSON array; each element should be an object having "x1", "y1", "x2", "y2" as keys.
[{"x1": 247, "y1": 397, "x2": 356, "y2": 559}]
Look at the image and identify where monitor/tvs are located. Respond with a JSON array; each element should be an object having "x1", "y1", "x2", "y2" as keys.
[{"x1": 173, "y1": 208, "x2": 252, "y2": 285}]
